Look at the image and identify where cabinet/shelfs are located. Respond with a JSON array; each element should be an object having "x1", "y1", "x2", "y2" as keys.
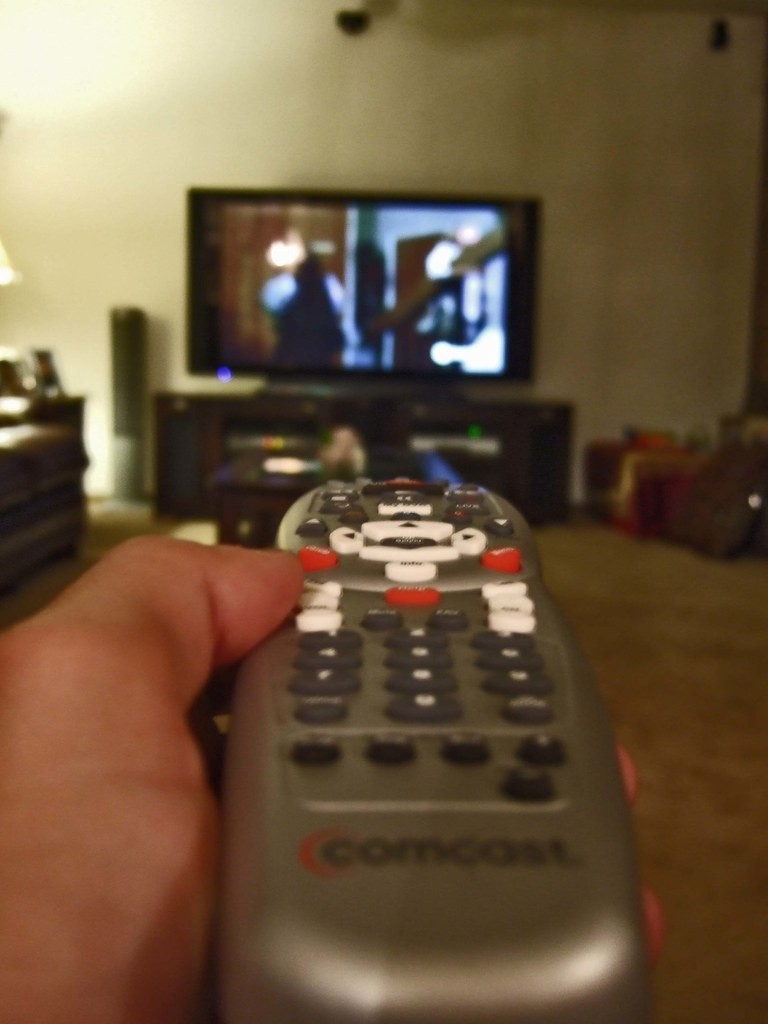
[{"x1": 150, "y1": 388, "x2": 575, "y2": 547}]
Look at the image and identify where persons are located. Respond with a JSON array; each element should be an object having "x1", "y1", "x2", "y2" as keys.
[{"x1": 0, "y1": 535, "x2": 666, "y2": 1024}]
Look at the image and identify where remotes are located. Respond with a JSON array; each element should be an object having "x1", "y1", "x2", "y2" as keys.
[{"x1": 209, "y1": 470, "x2": 656, "y2": 1023}]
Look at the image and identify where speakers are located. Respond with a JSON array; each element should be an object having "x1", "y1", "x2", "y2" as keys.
[{"x1": 111, "y1": 306, "x2": 152, "y2": 506}]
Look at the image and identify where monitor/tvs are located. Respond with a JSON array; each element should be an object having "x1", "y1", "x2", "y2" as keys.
[{"x1": 183, "y1": 188, "x2": 542, "y2": 402}]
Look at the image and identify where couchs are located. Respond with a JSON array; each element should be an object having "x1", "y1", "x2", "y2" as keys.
[{"x1": 1, "y1": 357, "x2": 89, "y2": 584}]
